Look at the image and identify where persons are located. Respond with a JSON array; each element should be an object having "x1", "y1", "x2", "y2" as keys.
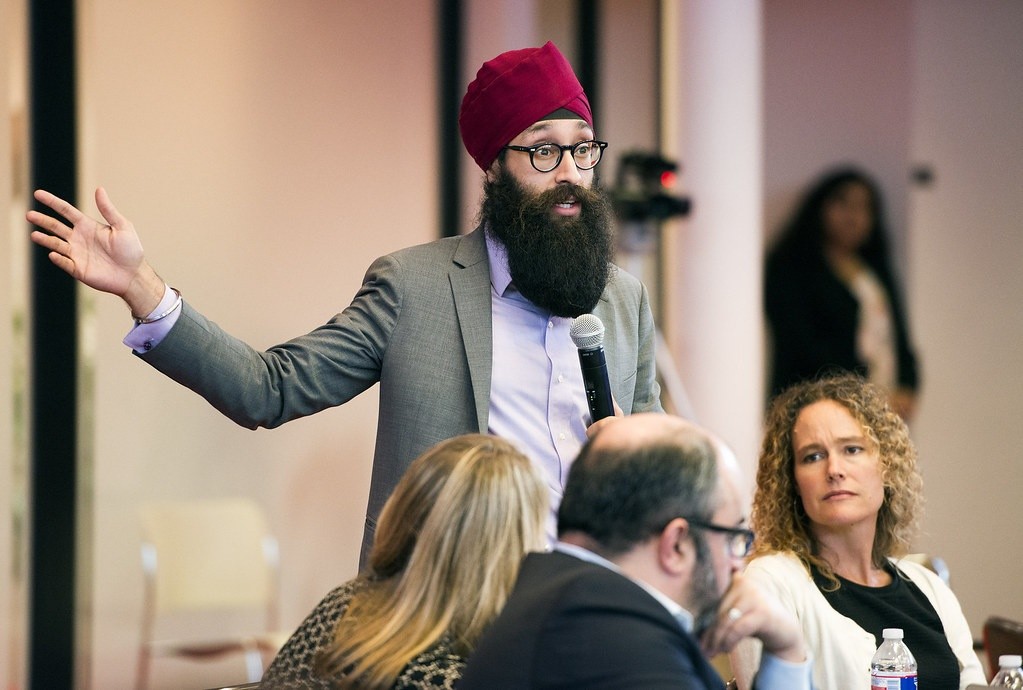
[
  {"x1": 27, "y1": 41, "x2": 665, "y2": 575},
  {"x1": 258, "y1": 432, "x2": 544, "y2": 690},
  {"x1": 744, "y1": 371, "x2": 988, "y2": 690},
  {"x1": 763, "y1": 162, "x2": 920, "y2": 420},
  {"x1": 452, "y1": 411, "x2": 812, "y2": 690}
]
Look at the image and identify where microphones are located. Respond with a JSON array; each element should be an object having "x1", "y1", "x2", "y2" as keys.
[{"x1": 569, "y1": 314, "x2": 616, "y2": 427}]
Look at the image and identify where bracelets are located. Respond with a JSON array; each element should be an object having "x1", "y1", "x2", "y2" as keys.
[{"x1": 131, "y1": 287, "x2": 181, "y2": 324}]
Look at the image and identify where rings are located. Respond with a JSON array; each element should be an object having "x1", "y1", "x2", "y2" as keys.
[{"x1": 726, "y1": 607, "x2": 741, "y2": 621}]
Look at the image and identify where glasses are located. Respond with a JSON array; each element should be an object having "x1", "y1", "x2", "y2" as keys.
[
  {"x1": 502, "y1": 140, "x2": 608, "y2": 173},
  {"x1": 653, "y1": 516, "x2": 755, "y2": 558}
]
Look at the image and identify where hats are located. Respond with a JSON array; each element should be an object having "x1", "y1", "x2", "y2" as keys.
[
  {"x1": 604, "y1": 151, "x2": 689, "y2": 220},
  {"x1": 458, "y1": 40, "x2": 594, "y2": 172}
]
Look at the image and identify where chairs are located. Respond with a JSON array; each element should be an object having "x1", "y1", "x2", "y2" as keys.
[{"x1": 127, "y1": 503, "x2": 297, "y2": 690}]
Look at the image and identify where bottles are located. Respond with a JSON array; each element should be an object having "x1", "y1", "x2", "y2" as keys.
[
  {"x1": 990, "y1": 655, "x2": 1023, "y2": 690},
  {"x1": 871, "y1": 628, "x2": 917, "y2": 690}
]
